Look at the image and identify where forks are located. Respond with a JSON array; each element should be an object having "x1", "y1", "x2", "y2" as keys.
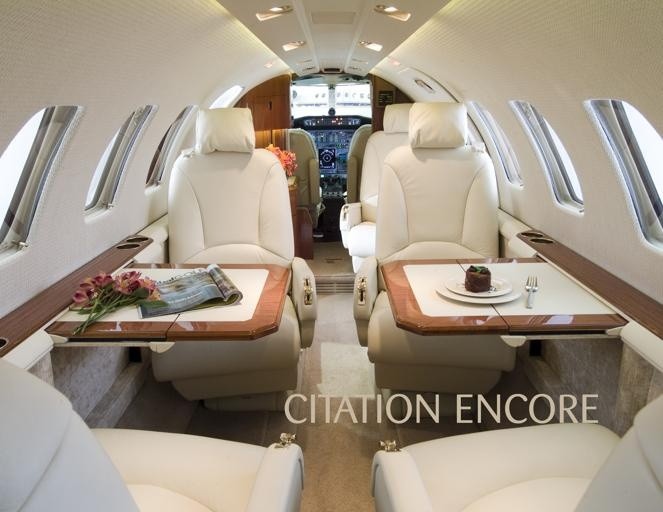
[{"x1": 524, "y1": 275, "x2": 543, "y2": 309}]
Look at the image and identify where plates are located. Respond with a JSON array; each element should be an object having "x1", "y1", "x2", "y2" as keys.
[
  {"x1": 444, "y1": 273, "x2": 514, "y2": 299},
  {"x1": 434, "y1": 279, "x2": 524, "y2": 306}
]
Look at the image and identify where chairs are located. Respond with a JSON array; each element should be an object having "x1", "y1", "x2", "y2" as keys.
[
  {"x1": 370, "y1": 390, "x2": 663, "y2": 512},
  {"x1": 0, "y1": 356, "x2": 305, "y2": 512}
]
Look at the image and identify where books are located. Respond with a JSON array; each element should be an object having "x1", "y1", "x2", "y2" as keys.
[{"x1": 133, "y1": 264, "x2": 242, "y2": 319}]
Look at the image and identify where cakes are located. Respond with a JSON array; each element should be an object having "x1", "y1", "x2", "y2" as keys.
[{"x1": 465, "y1": 266, "x2": 491, "y2": 292}]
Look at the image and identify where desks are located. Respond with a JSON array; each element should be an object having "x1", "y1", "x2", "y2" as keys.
[
  {"x1": 46, "y1": 262, "x2": 293, "y2": 354},
  {"x1": 380, "y1": 256, "x2": 628, "y2": 350}
]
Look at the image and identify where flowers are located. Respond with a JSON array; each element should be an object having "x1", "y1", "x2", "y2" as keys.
[{"x1": 66, "y1": 271, "x2": 159, "y2": 337}]
